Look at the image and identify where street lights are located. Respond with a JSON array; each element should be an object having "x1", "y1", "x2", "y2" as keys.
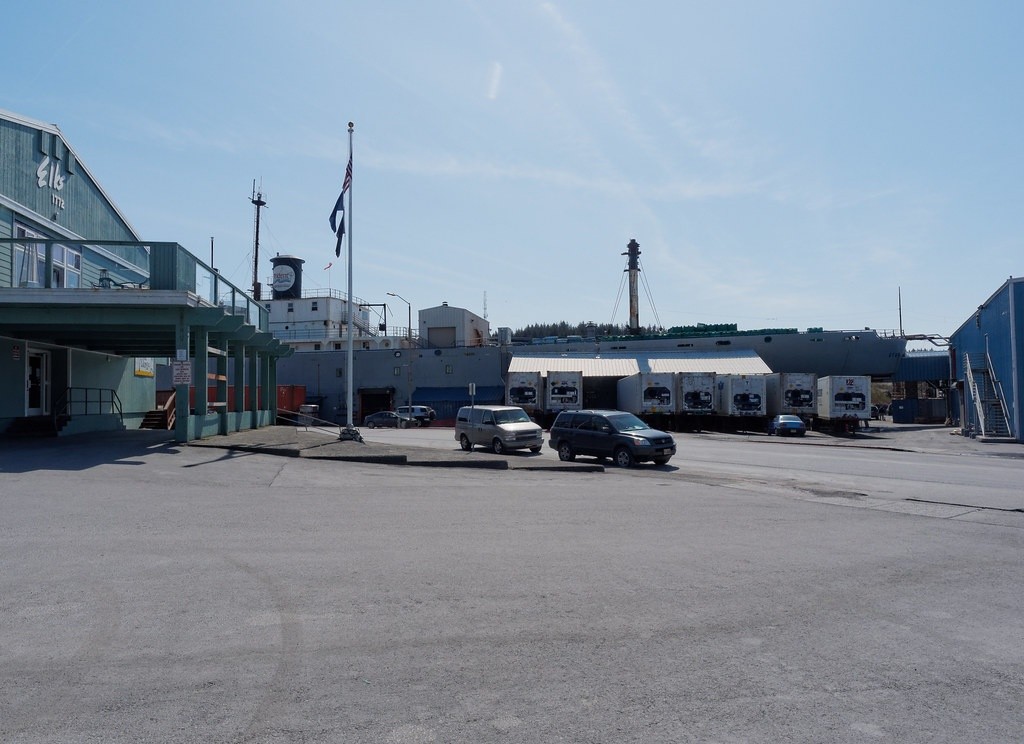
[{"x1": 386, "y1": 292, "x2": 411, "y2": 342}]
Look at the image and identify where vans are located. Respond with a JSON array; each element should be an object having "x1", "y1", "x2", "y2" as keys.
[{"x1": 454, "y1": 405, "x2": 544, "y2": 454}]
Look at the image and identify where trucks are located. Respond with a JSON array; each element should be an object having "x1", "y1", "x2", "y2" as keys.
[
  {"x1": 617, "y1": 371, "x2": 872, "y2": 432},
  {"x1": 504, "y1": 370, "x2": 585, "y2": 425}
]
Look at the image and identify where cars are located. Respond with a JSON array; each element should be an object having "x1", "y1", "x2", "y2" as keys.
[
  {"x1": 767, "y1": 414, "x2": 806, "y2": 437},
  {"x1": 363, "y1": 411, "x2": 422, "y2": 430}
]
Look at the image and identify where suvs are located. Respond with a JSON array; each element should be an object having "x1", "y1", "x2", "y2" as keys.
[
  {"x1": 548, "y1": 409, "x2": 677, "y2": 468},
  {"x1": 395, "y1": 405, "x2": 437, "y2": 427}
]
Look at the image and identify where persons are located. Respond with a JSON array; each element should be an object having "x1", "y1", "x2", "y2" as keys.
[
  {"x1": 864, "y1": 421, "x2": 870, "y2": 429},
  {"x1": 875, "y1": 403, "x2": 888, "y2": 421}
]
